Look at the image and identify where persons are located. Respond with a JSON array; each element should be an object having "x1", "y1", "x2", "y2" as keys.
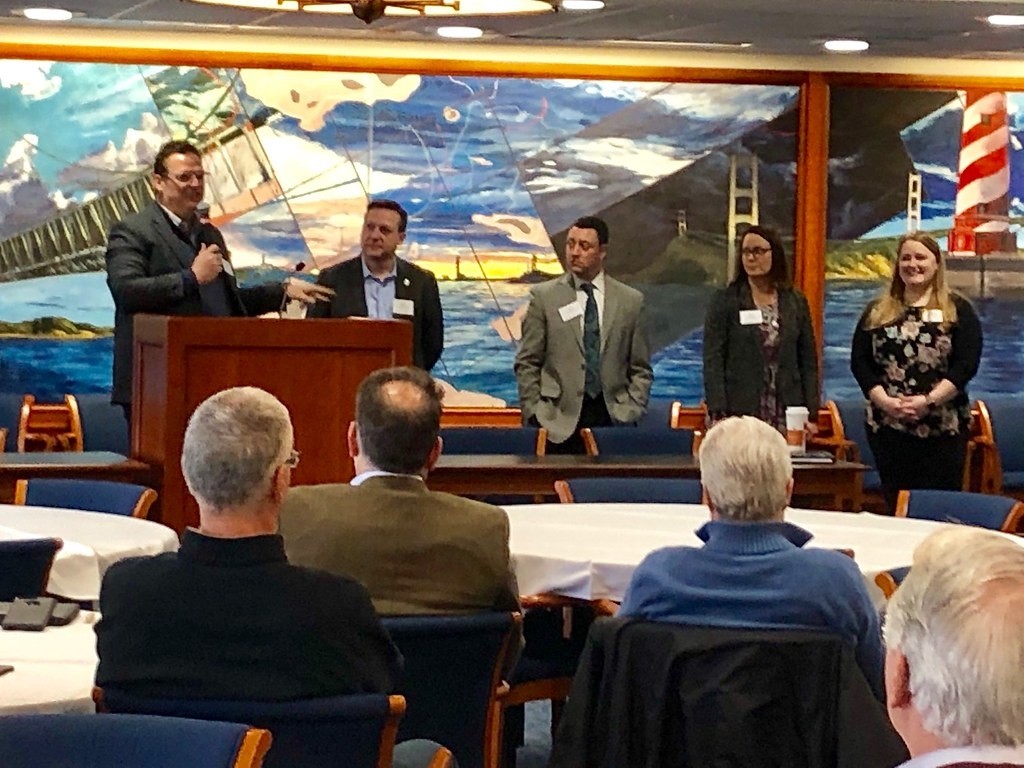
[
  {"x1": 278, "y1": 367, "x2": 524, "y2": 676},
  {"x1": 94, "y1": 386, "x2": 454, "y2": 768},
  {"x1": 305, "y1": 200, "x2": 444, "y2": 374},
  {"x1": 513, "y1": 215, "x2": 653, "y2": 454},
  {"x1": 884, "y1": 525, "x2": 1024, "y2": 768},
  {"x1": 850, "y1": 232, "x2": 982, "y2": 517},
  {"x1": 105, "y1": 141, "x2": 337, "y2": 439},
  {"x1": 618, "y1": 414, "x2": 885, "y2": 707},
  {"x1": 703, "y1": 227, "x2": 820, "y2": 436}
]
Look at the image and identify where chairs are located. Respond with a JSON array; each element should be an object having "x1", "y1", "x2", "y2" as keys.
[{"x1": 0, "y1": 392, "x2": 1024, "y2": 768}]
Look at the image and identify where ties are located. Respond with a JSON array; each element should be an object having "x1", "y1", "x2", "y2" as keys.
[
  {"x1": 580, "y1": 283, "x2": 602, "y2": 397},
  {"x1": 179, "y1": 220, "x2": 192, "y2": 239}
]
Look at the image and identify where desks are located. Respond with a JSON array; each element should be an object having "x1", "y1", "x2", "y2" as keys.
[
  {"x1": 499, "y1": 503, "x2": 954, "y2": 602},
  {"x1": 428, "y1": 455, "x2": 876, "y2": 506},
  {"x1": 0, "y1": 606, "x2": 104, "y2": 718},
  {"x1": 0, "y1": 506, "x2": 181, "y2": 603},
  {"x1": 0, "y1": 450, "x2": 152, "y2": 506}
]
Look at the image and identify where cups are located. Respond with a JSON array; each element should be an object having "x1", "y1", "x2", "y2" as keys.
[{"x1": 786, "y1": 405, "x2": 809, "y2": 458}]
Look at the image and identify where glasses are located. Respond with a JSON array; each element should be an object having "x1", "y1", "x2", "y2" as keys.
[
  {"x1": 281, "y1": 450, "x2": 301, "y2": 470},
  {"x1": 165, "y1": 172, "x2": 208, "y2": 183},
  {"x1": 742, "y1": 246, "x2": 772, "y2": 256}
]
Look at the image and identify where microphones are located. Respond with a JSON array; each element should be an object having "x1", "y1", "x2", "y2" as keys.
[
  {"x1": 196, "y1": 201, "x2": 214, "y2": 247},
  {"x1": 280, "y1": 262, "x2": 306, "y2": 318}
]
